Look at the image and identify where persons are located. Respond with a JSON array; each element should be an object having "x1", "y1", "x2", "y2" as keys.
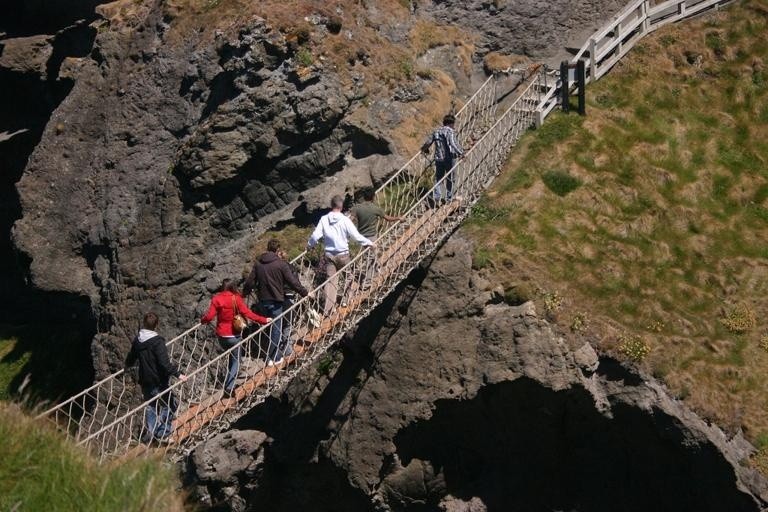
[
  {"x1": 305, "y1": 195, "x2": 377, "y2": 317},
  {"x1": 347, "y1": 188, "x2": 405, "y2": 290},
  {"x1": 422, "y1": 114, "x2": 463, "y2": 203},
  {"x1": 201, "y1": 278, "x2": 272, "y2": 397},
  {"x1": 241, "y1": 238, "x2": 315, "y2": 366},
  {"x1": 125, "y1": 312, "x2": 190, "y2": 444}
]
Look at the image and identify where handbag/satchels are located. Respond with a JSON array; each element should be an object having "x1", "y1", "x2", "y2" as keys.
[{"x1": 233, "y1": 314, "x2": 249, "y2": 331}]
